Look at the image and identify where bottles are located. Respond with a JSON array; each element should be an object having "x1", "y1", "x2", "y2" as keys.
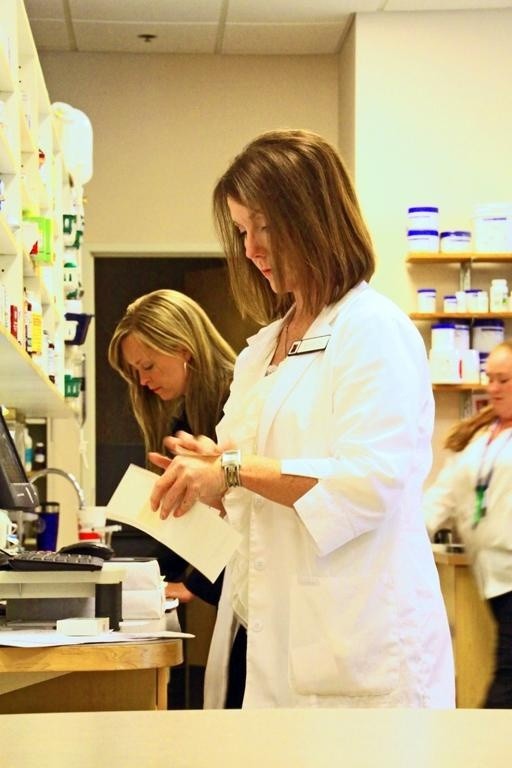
[{"x1": 405, "y1": 205, "x2": 511, "y2": 384}]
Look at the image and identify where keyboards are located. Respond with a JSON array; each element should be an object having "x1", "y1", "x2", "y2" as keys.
[{"x1": 7, "y1": 548, "x2": 105, "y2": 570}]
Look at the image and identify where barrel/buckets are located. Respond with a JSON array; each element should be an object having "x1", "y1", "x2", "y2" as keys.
[{"x1": 77, "y1": 506, "x2": 106, "y2": 546}]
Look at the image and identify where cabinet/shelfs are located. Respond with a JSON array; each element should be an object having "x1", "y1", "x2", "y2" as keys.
[
  {"x1": 1, "y1": 0, "x2": 87, "y2": 425},
  {"x1": 433, "y1": 549, "x2": 500, "y2": 708},
  {"x1": 405, "y1": 250, "x2": 512, "y2": 394}
]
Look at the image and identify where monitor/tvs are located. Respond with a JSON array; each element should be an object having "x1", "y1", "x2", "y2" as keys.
[{"x1": 0, "y1": 408, "x2": 42, "y2": 513}]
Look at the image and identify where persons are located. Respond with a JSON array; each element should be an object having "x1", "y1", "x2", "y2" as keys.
[
  {"x1": 422, "y1": 336, "x2": 512, "y2": 709},
  {"x1": 108, "y1": 288, "x2": 248, "y2": 709},
  {"x1": 150, "y1": 129, "x2": 458, "y2": 709}
]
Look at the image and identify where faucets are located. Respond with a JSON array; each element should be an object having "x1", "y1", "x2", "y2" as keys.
[{"x1": 29, "y1": 468, "x2": 85, "y2": 512}]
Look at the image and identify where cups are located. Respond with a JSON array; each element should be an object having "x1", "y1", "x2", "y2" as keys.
[
  {"x1": 74, "y1": 509, "x2": 107, "y2": 542},
  {"x1": 33, "y1": 501, "x2": 59, "y2": 553}
]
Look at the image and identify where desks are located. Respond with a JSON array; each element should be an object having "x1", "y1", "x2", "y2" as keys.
[{"x1": 0, "y1": 636, "x2": 186, "y2": 712}]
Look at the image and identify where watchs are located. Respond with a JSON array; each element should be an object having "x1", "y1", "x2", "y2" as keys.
[{"x1": 221, "y1": 450, "x2": 242, "y2": 488}]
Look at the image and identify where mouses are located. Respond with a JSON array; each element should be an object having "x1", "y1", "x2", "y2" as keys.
[{"x1": 60, "y1": 541, "x2": 114, "y2": 560}]
